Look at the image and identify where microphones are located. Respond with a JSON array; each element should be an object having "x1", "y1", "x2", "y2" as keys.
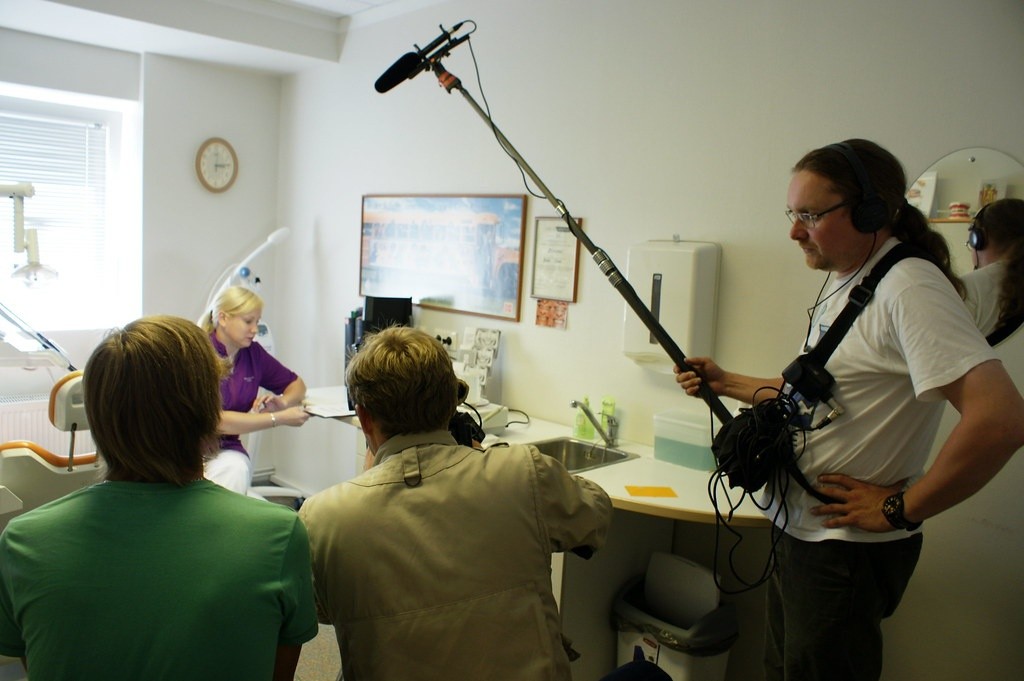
[{"x1": 374, "y1": 21, "x2": 464, "y2": 93}]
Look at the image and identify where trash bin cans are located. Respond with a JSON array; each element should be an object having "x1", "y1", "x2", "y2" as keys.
[{"x1": 610, "y1": 551, "x2": 738, "y2": 681}]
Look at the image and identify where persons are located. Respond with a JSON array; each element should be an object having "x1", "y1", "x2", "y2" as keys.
[
  {"x1": 0, "y1": 315, "x2": 318, "y2": 681},
  {"x1": 296, "y1": 323, "x2": 612, "y2": 681},
  {"x1": 672, "y1": 139, "x2": 1024, "y2": 681},
  {"x1": 199, "y1": 287, "x2": 312, "y2": 503},
  {"x1": 952, "y1": 198, "x2": 1024, "y2": 346}
]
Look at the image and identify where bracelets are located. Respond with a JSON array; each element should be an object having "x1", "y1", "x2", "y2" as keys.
[{"x1": 270, "y1": 414, "x2": 276, "y2": 428}]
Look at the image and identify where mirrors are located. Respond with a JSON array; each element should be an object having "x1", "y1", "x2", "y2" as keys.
[{"x1": 904, "y1": 146, "x2": 1024, "y2": 352}]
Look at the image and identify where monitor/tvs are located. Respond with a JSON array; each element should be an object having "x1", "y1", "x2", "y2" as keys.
[{"x1": 363, "y1": 298, "x2": 413, "y2": 340}]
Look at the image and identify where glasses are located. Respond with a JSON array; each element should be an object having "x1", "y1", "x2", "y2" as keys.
[
  {"x1": 785, "y1": 201, "x2": 848, "y2": 229},
  {"x1": 965, "y1": 240, "x2": 972, "y2": 250}
]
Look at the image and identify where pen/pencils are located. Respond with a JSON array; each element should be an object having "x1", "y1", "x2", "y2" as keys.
[{"x1": 301, "y1": 403, "x2": 307, "y2": 410}]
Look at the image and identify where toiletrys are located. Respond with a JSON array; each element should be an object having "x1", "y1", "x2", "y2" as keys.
[
  {"x1": 601, "y1": 390, "x2": 616, "y2": 440},
  {"x1": 575, "y1": 392, "x2": 594, "y2": 440}
]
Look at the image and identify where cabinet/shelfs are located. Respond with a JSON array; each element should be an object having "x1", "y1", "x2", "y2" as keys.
[{"x1": 247, "y1": 385, "x2": 773, "y2": 680}]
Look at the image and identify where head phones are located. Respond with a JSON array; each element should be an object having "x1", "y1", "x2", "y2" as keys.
[{"x1": 968, "y1": 202, "x2": 992, "y2": 251}]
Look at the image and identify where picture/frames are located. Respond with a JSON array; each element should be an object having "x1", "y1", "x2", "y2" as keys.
[{"x1": 358, "y1": 194, "x2": 529, "y2": 321}]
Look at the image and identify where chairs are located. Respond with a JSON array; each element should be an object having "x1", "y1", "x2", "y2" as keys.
[{"x1": 0, "y1": 370, "x2": 109, "y2": 531}]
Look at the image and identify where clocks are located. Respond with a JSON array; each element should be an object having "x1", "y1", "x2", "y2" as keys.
[{"x1": 195, "y1": 138, "x2": 239, "y2": 192}]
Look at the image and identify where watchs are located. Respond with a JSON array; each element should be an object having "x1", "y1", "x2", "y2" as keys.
[{"x1": 881, "y1": 491, "x2": 924, "y2": 531}]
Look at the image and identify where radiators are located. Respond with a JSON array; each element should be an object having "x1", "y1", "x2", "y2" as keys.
[{"x1": 0, "y1": 399, "x2": 96, "y2": 458}]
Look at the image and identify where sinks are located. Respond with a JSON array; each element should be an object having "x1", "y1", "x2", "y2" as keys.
[{"x1": 518, "y1": 437, "x2": 641, "y2": 474}]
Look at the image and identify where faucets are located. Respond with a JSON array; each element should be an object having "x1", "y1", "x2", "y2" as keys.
[{"x1": 569, "y1": 400, "x2": 618, "y2": 447}]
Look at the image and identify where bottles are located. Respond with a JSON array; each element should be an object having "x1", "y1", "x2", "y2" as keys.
[
  {"x1": 572, "y1": 394, "x2": 595, "y2": 440},
  {"x1": 600, "y1": 395, "x2": 615, "y2": 435}
]
